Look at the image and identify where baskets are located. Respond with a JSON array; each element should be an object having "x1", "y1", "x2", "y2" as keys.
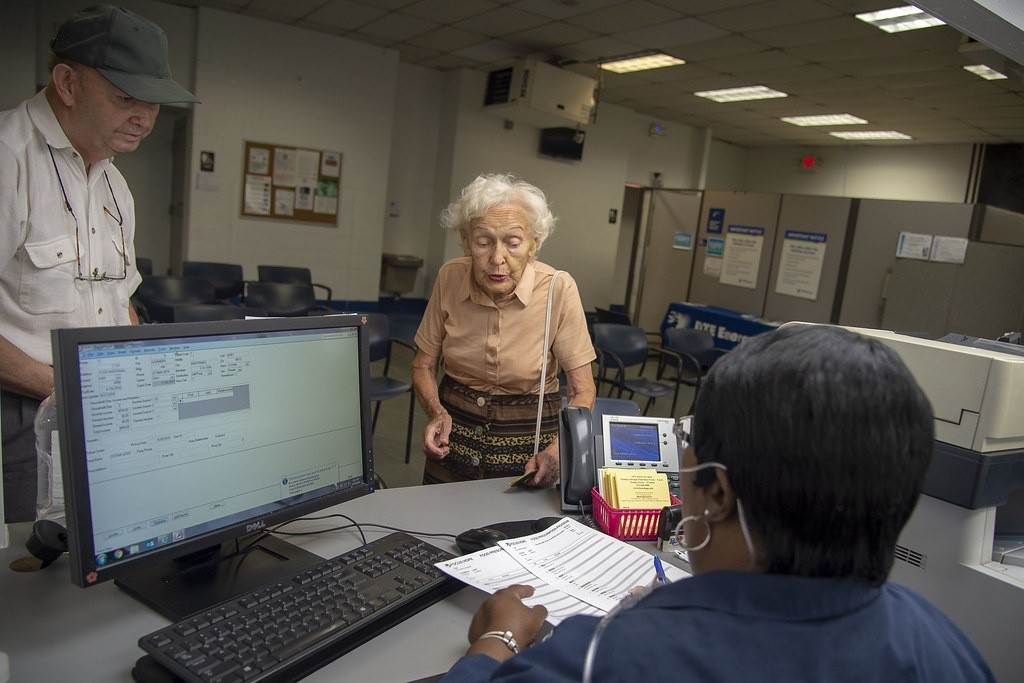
[{"x1": 590, "y1": 486, "x2": 682, "y2": 541}]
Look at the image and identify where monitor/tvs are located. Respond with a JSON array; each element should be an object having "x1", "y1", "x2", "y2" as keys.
[
  {"x1": 49, "y1": 316, "x2": 375, "y2": 624},
  {"x1": 608, "y1": 422, "x2": 663, "y2": 462}
]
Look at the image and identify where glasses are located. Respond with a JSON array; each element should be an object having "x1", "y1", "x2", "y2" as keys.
[
  {"x1": 65, "y1": 202, "x2": 127, "y2": 281},
  {"x1": 671, "y1": 415, "x2": 694, "y2": 447}
]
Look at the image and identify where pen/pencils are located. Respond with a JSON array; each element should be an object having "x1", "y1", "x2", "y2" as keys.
[{"x1": 654, "y1": 555, "x2": 667, "y2": 585}]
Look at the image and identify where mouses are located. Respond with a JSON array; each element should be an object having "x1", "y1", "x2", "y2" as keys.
[{"x1": 456, "y1": 528, "x2": 510, "y2": 555}]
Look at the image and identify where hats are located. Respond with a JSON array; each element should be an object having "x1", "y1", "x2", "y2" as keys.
[{"x1": 49, "y1": 4, "x2": 201, "y2": 105}]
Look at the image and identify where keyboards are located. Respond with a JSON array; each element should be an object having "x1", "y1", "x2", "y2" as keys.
[{"x1": 138, "y1": 531, "x2": 462, "y2": 683}]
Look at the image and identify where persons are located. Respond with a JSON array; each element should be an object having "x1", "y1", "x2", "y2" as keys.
[
  {"x1": 437, "y1": 326, "x2": 996, "y2": 683},
  {"x1": 0, "y1": 4, "x2": 201, "y2": 524},
  {"x1": 410, "y1": 172, "x2": 595, "y2": 485}
]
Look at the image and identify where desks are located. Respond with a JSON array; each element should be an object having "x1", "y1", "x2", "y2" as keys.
[
  {"x1": 0, "y1": 476, "x2": 694, "y2": 683},
  {"x1": 658, "y1": 302, "x2": 779, "y2": 375}
]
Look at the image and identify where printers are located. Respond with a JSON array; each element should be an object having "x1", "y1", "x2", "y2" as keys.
[{"x1": 776, "y1": 321, "x2": 1023, "y2": 683}]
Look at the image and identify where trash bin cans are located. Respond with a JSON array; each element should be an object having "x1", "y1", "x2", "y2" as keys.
[{"x1": 381, "y1": 254, "x2": 424, "y2": 294}]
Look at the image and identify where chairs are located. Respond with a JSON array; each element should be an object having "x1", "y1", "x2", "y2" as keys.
[
  {"x1": 182, "y1": 260, "x2": 254, "y2": 304},
  {"x1": 359, "y1": 313, "x2": 418, "y2": 465},
  {"x1": 247, "y1": 282, "x2": 311, "y2": 317},
  {"x1": 257, "y1": 264, "x2": 332, "y2": 311},
  {"x1": 136, "y1": 258, "x2": 153, "y2": 276},
  {"x1": 557, "y1": 305, "x2": 731, "y2": 419},
  {"x1": 136, "y1": 276, "x2": 215, "y2": 305},
  {"x1": 172, "y1": 304, "x2": 269, "y2": 324}
]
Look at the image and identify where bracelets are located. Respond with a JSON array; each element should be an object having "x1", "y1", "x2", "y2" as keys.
[{"x1": 478, "y1": 631, "x2": 519, "y2": 654}]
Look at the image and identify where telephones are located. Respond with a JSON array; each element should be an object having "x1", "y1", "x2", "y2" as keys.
[{"x1": 558, "y1": 406, "x2": 682, "y2": 511}]
